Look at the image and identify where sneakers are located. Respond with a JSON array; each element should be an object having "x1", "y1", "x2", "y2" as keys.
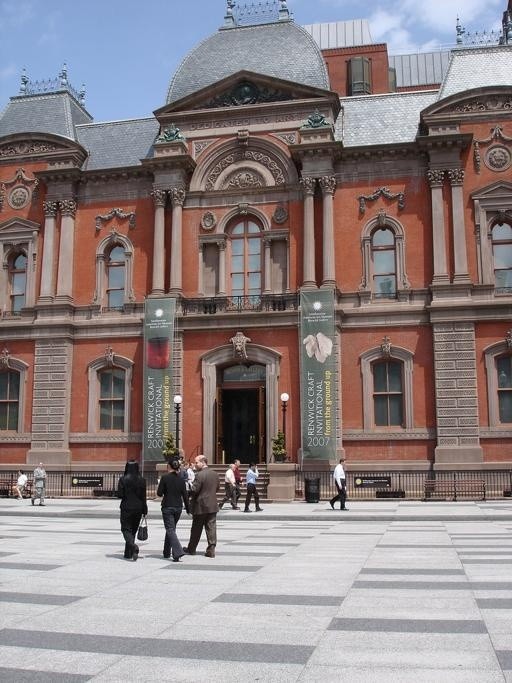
[
  {"x1": 330, "y1": 502, "x2": 335, "y2": 510},
  {"x1": 232, "y1": 507, "x2": 240, "y2": 510},
  {"x1": 183, "y1": 547, "x2": 191, "y2": 553},
  {"x1": 206, "y1": 553, "x2": 214, "y2": 558},
  {"x1": 244, "y1": 510, "x2": 253, "y2": 512},
  {"x1": 256, "y1": 509, "x2": 263, "y2": 511},
  {"x1": 18, "y1": 496, "x2": 23, "y2": 500},
  {"x1": 341, "y1": 507, "x2": 348, "y2": 510},
  {"x1": 219, "y1": 503, "x2": 222, "y2": 509},
  {"x1": 39, "y1": 504, "x2": 44, "y2": 506},
  {"x1": 32, "y1": 499, "x2": 34, "y2": 505}
]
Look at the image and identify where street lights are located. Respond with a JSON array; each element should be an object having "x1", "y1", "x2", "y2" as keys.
[
  {"x1": 173, "y1": 395, "x2": 183, "y2": 458},
  {"x1": 280, "y1": 392, "x2": 289, "y2": 450}
]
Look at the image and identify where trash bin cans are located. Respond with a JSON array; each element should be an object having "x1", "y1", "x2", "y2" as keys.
[{"x1": 305, "y1": 478, "x2": 321, "y2": 503}]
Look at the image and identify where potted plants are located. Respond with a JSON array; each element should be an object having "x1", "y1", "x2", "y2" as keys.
[
  {"x1": 271, "y1": 431, "x2": 288, "y2": 463},
  {"x1": 161, "y1": 432, "x2": 179, "y2": 464}
]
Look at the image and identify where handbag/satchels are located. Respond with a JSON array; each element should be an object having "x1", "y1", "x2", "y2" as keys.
[{"x1": 138, "y1": 527, "x2": 148, "y2": 539}]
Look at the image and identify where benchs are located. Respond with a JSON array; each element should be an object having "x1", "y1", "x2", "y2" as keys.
[
  {"x1": 424, "y1": 480, "x2": 486, "y2": 501},
  {"x1": 0, "y1": 479, "x2": 34, "y2": 498}
]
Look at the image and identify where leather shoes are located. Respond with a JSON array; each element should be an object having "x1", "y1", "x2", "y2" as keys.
[{"x1": 132, "y1": 547, "x2": 139, "y2": 560}]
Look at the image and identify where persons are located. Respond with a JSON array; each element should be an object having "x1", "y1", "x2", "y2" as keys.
[
  {"x1": 329, "y1": 458, "x2": 349, "y2": 510},
  {"x1": 183, "y1": 454, "x2": 221, "y2": 558},
  {"x1": 114, "y1": 459, "x2": 148, "y2": 560},
  {"x1": 157, "y1": 459, "x2": 191, "y2": 562},
  {"x1": 32, "y1": 461, "x2": 48, "y2": 505},
  {"x1": 16, "y1": 469, "x2": 29, "y2": 499},
  {"x1": 176, "y1": 455, "x2": 242, "y2": 515},
  {"x1": 242, "y1": 463, "x2": 264, "y2": 512}
]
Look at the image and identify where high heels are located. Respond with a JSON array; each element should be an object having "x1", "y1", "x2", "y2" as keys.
[
  {"x1": 163, "y1": 554, "x2": 168, "y2": 557},
  {"x1": 173, "y1": 556, "x2": 181, "y2": 562}
]
